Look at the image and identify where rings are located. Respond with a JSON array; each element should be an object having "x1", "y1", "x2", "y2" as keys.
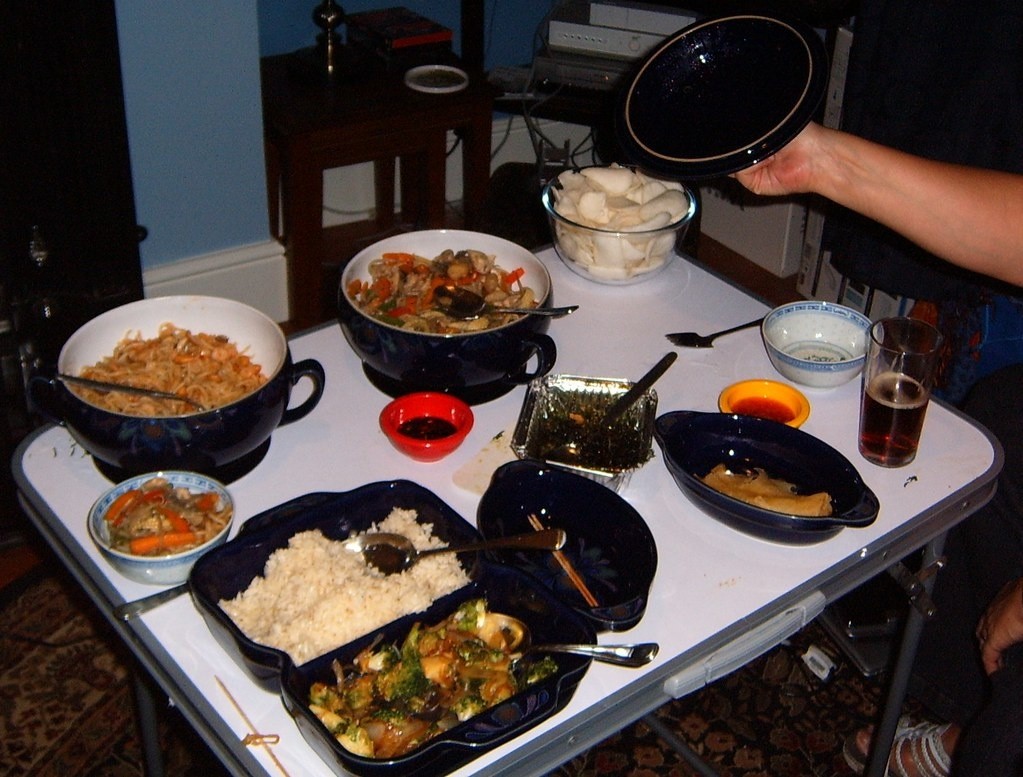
[{"x1": 980, "y1": 627, "x2": 987, "y2": 639}]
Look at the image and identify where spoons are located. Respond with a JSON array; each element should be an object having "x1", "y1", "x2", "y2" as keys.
[
  {"x1": 434, "y1": 286, "x2": 580, "y2": 320},
  {"x1": 344, "y1": 529, "x2": 566, "y2": 577},
  {"x1": 490, "y1": 613, "x2": 660, "y2": 668},
  {"x1": 543, "y1": 352, "x2": 677, "y2": 459}
]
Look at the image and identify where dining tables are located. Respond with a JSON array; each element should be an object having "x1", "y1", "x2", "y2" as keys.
[{"x1": 18, "y1": 238, "x2": 1007, "y2": 777}]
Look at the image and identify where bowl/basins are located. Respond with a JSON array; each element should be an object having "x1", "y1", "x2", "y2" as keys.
[
  {"x1": 187, "y1": 478, "x2": 597, "y2": 777},
  {"x1": 336, "y1": 228, "x2": 556, "y2": 407},
  {"x1": 29, "y1": 295, "x2": 325, "y2": 487},
  {"x1": 541, "y1": 163, "x2": 696, "y2": 287},
  {"x1": 379, "y1": 392, "x2": 475, "y2": 462},
  {"x1": 512, "y1": 375, "x2": 658, "y2": 496},
  {"x1": 476, "y1": 459, "x2": 658, "y2": 633},
  {"x1": 718, "y1": 380, "x2": 810, "y2": 430},
  {"x1": 761, "y1": 302, "x2": 875, "y2": 389},
  {"x1": 86, "y1": 471, "x2": 236, "y2": 586},
  {"x1": 653, "y1": 410, "x2": 879, "y2": 546}
]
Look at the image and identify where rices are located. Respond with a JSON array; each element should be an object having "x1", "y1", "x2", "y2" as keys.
[{"x1": 217, "y1": 506, "x2": 471, "y2": 665}]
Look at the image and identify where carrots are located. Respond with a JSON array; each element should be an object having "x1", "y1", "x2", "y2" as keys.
[{"x1": 102, "y1": 489, "x2": 231, "y2": 553}]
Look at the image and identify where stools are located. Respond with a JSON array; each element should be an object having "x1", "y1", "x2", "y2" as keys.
[{"x1": 260, "y1": 49, "x2": 507, "y2": 325}]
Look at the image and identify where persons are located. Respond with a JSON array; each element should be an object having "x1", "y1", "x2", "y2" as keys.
[{"x1": 731, "y1": 121, "x2": 1023, "y2": 777}]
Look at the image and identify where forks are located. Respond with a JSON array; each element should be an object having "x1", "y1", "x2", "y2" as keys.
[{"x1": 665, "y1": 316, "x2": 768, "y2": 348}]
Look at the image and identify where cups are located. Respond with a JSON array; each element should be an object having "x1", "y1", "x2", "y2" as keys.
[{"x1": 857, "y1": 316, "x2": 942, "y2": 468}]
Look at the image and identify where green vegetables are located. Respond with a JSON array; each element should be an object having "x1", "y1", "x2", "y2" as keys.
[{"x1": 310, "y1": 599, "x2": 558, "y2": 750}]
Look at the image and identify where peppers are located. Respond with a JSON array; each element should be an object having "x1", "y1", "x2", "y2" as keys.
[{"x1": 351, "y1": 252, "x2": 525, "y2": 333}]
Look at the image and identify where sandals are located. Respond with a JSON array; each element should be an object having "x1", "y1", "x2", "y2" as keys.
[{"x1": 841, "y1": 715, "x2": 959, "y2": 777}]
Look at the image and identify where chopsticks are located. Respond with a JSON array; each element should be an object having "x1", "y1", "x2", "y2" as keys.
[{"x1": 527, "y1": 514, "x2": 597, "y2": 607}]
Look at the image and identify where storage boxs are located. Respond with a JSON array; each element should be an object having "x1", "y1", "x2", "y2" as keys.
[{"x1": 347, "y1": 4, "x2": 455, "y2": 69}]
